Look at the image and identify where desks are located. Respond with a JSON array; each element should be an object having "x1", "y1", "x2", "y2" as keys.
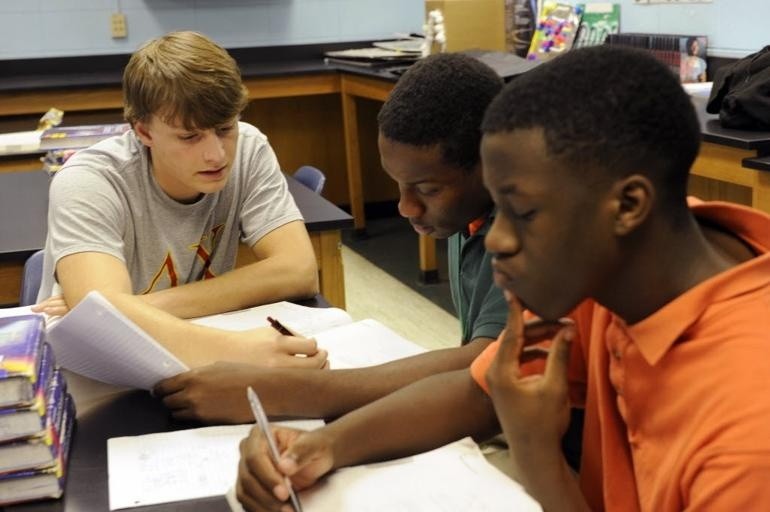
[{"x1": 0, "y1": 37, "x2": 770, "y2": 511}]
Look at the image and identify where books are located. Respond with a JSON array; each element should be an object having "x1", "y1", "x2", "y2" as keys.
[
  {"x1": 0, "y1": 315, "x2": 45, "y2": 412},
  {"x1": 324, "y1": 47, "x2": 419, "y2": 63},
  {"x1": 0, "y1": 368, "x2": 72, "y2": 475},
  {"x1": 608, "y1": 30, "x2": 710, "y2": 84},
  {"x1": 0, "y1": 390, "x2": 78, "y2": 506},
  {"x1": 0, "y1": 339, "x2": 58, "y2": 442},
  {"x1": 35, "y1": 120, "x2": 133, "y2": 149},
  {"x1": 369, "y1": 38, "x2": 422, "y2": 51}
]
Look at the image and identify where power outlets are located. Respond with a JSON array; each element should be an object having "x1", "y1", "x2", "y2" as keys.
[{"x1": 111, "y1": 14, "x2": 128, "y2": 38}]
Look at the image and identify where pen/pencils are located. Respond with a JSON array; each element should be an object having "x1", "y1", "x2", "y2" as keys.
[
  {"x1": 246, "y1": 385, "x2": 302, "y2": 512},
  {"x1": 266, "y1": 316, "x2": 294, "y2": 335}
]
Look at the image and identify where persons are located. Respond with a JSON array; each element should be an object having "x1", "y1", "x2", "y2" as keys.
[
  {"x1": 155, "y1": 47, "x2": 528, "y2": 429},
  {"x1": 236, "y1": 40, "x2": 770, "y2": 511},
  {"x1": 30, "y1": 26, "x2": 333, "y2": 374}
]
